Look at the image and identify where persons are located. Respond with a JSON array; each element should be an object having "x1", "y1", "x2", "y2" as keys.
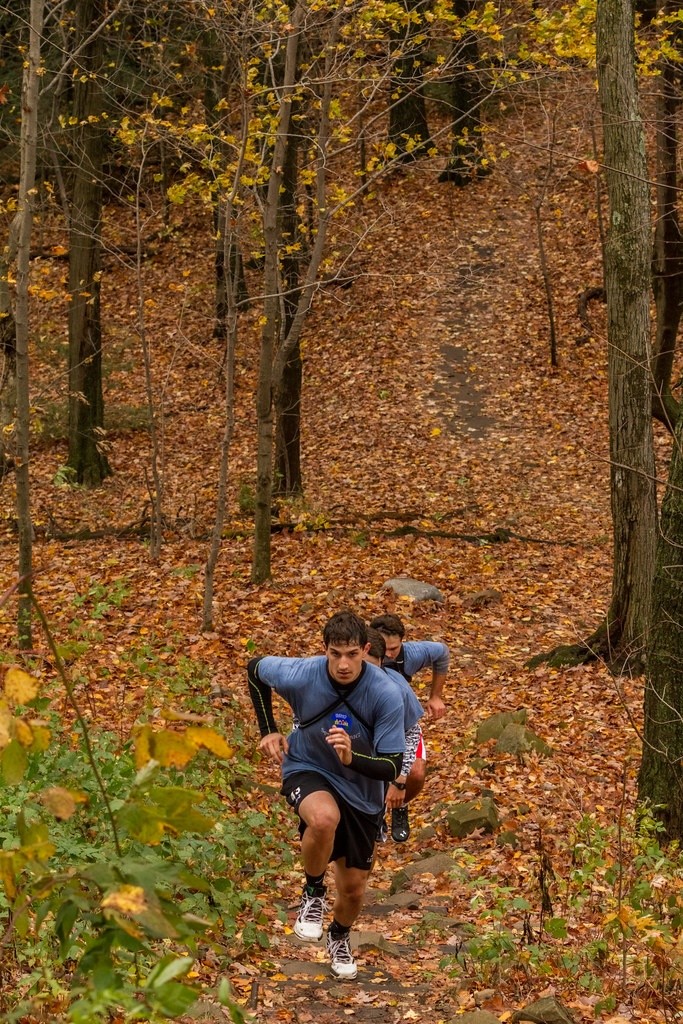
[
  {"x1": 360, "y1": 626, "x2": 424, "y2": 881},
  {"x1": 246, "y1": 609, "x2": 407, "y2": 981},
  {"x1": 367, "y1": 613, "x2": 451, "y2": 844}
]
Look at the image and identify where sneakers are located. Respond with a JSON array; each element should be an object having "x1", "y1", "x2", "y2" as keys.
[
  {"x1": 390, "y1": 804, "x2": 411, "y2": 843},
  {"x1": 326, "y1": 922, "x2": 358, "y2": 981},
  {"x1": 293, "y1": 884, "x2": 327, "y2": 943}
]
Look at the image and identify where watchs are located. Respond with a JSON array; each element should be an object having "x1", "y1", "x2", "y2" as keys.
[{"x1": 391, "y1": 781, "x2": 407, "y2": 790}]
